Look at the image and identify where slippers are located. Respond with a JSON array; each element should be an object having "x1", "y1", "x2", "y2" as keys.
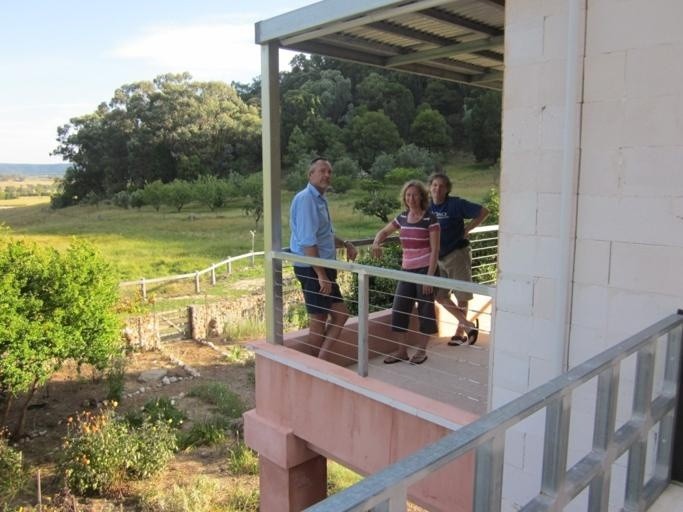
[
  {"x1": 448, "y1": 335, "x2": 467, "y2": 346},
  {"x1": 468, "y1": 319, "x2": 479, "y2": 345},
  {"x1": 411, "y1": 355, "x2": 427, "y2": 364},
  {"x1": 384, "y1": 354, "x2": 409, "y2": 365}
]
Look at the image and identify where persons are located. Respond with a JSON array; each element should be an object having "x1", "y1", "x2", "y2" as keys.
[
  {"x1": 289, "y1": 158, "x2": 357, "y2": 360},
  {"x1": 371, "y1": 181, "x2": 441, "y2": 365},
  {"x1": 424, "y1": 173, "x2": 489, "y2": 347}
]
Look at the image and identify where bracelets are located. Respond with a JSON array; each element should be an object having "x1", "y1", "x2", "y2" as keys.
[{"x1": 343, "y1": 239, "x2": 348, "y2": 246}]
